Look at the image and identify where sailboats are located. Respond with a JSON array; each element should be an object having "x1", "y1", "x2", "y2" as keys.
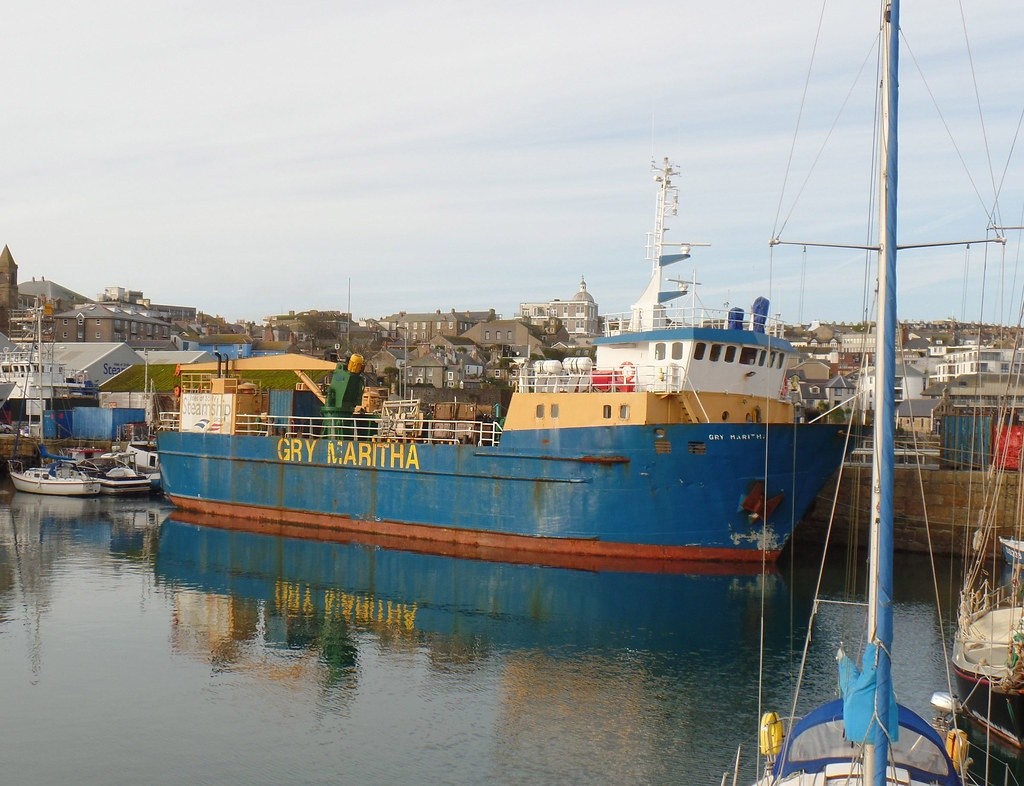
[
  {"x1": 7, "y1": 295, "x2": 102, "y2": 497},
  {"x1": 951, "y1": 111, "x2": 1024, "y2": 764},
  {"x1": 721, "y1": 0, "x2": 1019, "y2": 786}
]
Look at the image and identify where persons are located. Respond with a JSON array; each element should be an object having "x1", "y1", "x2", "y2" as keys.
[{"x1": 755, "y1": 406, "x2": 761, "y2": 423}]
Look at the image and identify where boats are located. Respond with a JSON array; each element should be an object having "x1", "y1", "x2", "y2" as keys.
[
  {"x1": 998, "y1": 536, "x2": 1024, "y2": 567},
  {"x1": 151, "y1": 513, "x2": 818, "y2": 660},
  {"x1": 76, "y1": 453, "x2": 151, "y2": 495},
  {"x1": 126, "y1": 442, "x2": 161, "y2": 488},
  {"x1": 153, "y1": 154, "x2": 873, "y2": 575}
]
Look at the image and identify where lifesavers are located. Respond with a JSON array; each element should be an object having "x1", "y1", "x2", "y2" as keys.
[{"x1": 618, "y1": 361, "x2": 635, "y2": 381}]
